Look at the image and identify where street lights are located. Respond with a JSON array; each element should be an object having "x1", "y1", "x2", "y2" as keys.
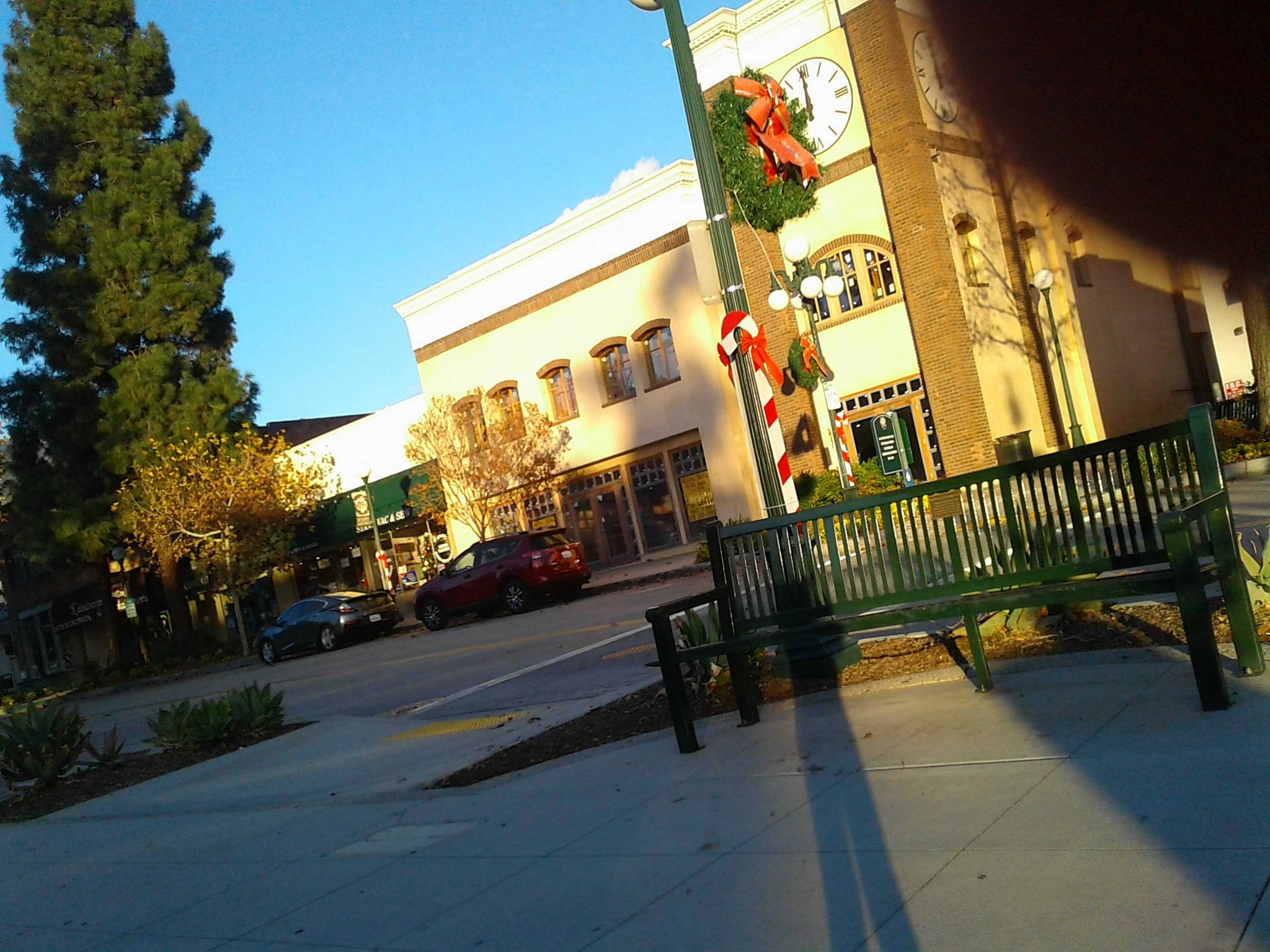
[
  {"x1": 1033, "y1": 268, "x2": 1086, "y2": 449},
  {"x1": 768, "y1": 234, "x2": 861, "y2": 501},
  {"x1": 357, "y1": 466, "x2": 398, "y2": 608}
]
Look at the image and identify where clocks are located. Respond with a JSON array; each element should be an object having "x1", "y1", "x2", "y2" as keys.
[
  {"x1": 777, "y1": 57, "x2": 852, "y2": 156},
  {"x1": 913, "y1": 30, "x2": 960, "y2": 123}
]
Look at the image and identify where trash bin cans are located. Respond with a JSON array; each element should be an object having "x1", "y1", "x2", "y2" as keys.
[{"x1": 994, "y1": 429, "x2": 1034, "y2": 466}]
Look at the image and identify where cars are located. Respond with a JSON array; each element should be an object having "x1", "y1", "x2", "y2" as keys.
[
  {"x1": 413, "y1": 527, "x2": 592, "y2": 632},
  {"x1": 258, "y1": 589, "x2": 400, "y2": 664}
]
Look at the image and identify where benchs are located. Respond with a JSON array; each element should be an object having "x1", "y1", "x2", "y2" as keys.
[{"x1": 644, "y1": 401, "x2": 1267, "y2": 755}]
